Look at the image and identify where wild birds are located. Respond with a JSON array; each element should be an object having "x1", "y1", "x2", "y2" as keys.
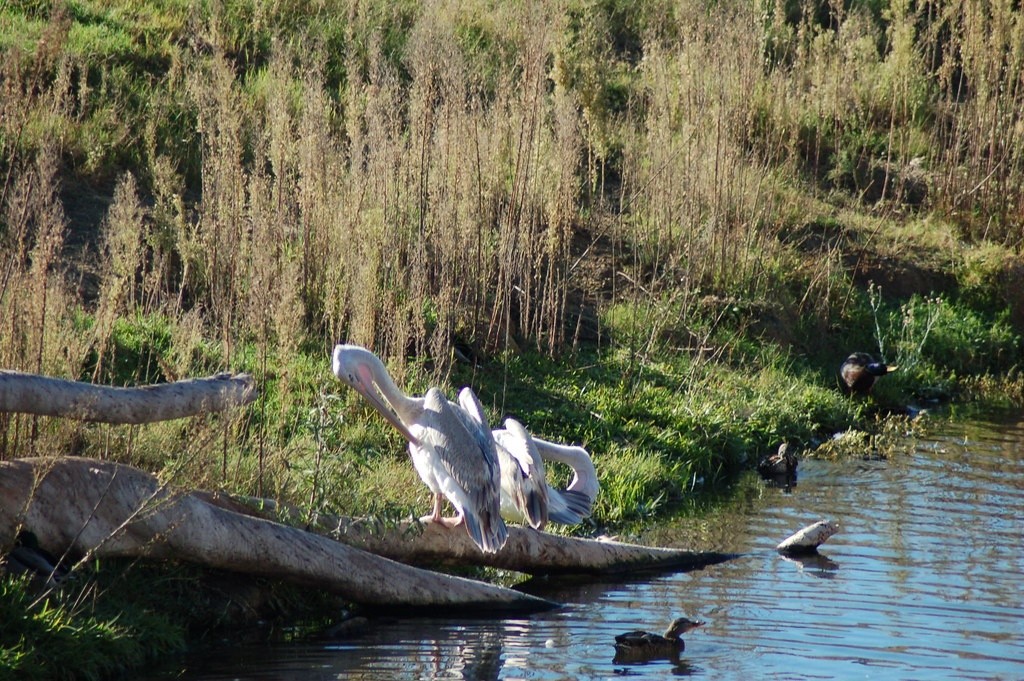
[
  {"x1": 840, "y1": 351, "x2": 900, "y2": 395},
  {"x1": 332, "y1": 343, "x2": 600, "y2": 555},
  {"x1": 756, "y1": 443, "x2": 800, "y2": 481},
  {"x1": 612, "y1": 616, "x2": 707, "y2": 653}
]
